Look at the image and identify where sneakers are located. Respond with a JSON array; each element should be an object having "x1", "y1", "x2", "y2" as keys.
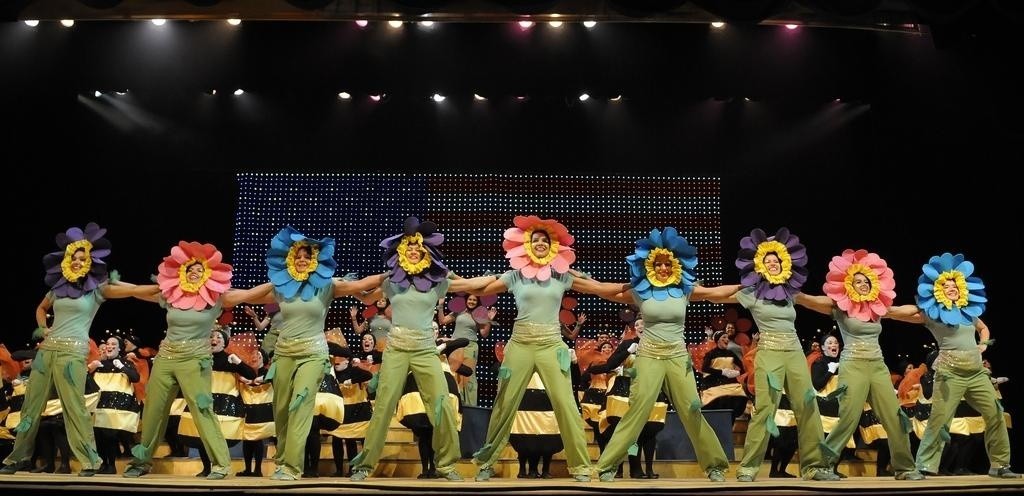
[
  {"x1": 572, "y1": 475, "x2": 593, "y2": 483},
  {"x1": 0, "y1": 460, "x2": 31, "y2": 474},
  {"x1": 78, "y1": 465, "x2": 104, "y2": 477},
  {"x1": 905, "y1": 473, "x2": 922, "y2": 480},
  {"x1": 999, "y1": 469, "x2": 1017, "y2": 478},
  {"x1": 827, "y1": 473, "x2": 840, "y2": 481},
  {"x1": 270, "y1": 472, "x2": 282, "y2": 480},
  {"x1": 350, "y1": 472, "x2": 367, "y2": 481},
  {"x1": 280, "y1": 473, "x2": 294, "y2": 481},
  {"x1": 445, "y1": 471, "x2": 466, "y2": 481},
  {"x1": 709, "y1": 471, "x2": 727, "y2": 482},
  {"x1": 206, "y1": 472, "x2": 225, "y2": 480},
  {"x1": 599, "y1": 472, "x2": 616, "y2": 482},
  {"x1": 813, "y1": 472, "x2": 830, "y2": 481},
  {"x1": 738, "y1": 476, "x2": 753, "y2": 482},
  {"x1": 475, "y1": 470, "x2": 491, "y2": 482},
  {"x1": 123, "y1": 467, "x2": 146, "y2": 477}
]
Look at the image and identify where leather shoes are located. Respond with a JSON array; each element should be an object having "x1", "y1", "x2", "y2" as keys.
[
  {"x1": 417, "y1": 472, "x2": 437, "y2": 479},
  {"x1": 301, "y1": 473, "x2": 311, "y2": 477},
  {"x1": 527, "y1": 473, "x2": 540, "y2": 479},
  {"x1": 516, "y1": 472, "x2": 528, "y2": 478},
  {"x1": 635, "y1": 474, "x2": 650, "y2": 479},
  {"x1": 250, "y1": 472, "x2": 262, "y2": 476},
  {"x1": 344, "y1": 472, "x2": 353, "y2": 477},
  {"x1": 648, "y1": 473, "x2": 660, "y2": 479},
  {"x1": 99, "y1": 464, "x2": 105, "y2": 473},
  {"x1": 614, "y1": 473, "x2": 624, "y2": 478},
  {"x1": 60, "y1": 464, "x2": 72, "y2": 473},
  {"x1": 786, "y1": 473, "x2": 796, "y2": 478},
  {"x1": 312, "y1": 472, "x2": 320, "y2": 477},
  {"x1": 834, "y1": 471, "x2": 848, "y2": 478},
  {"x1": 31, "y1": 465, "x2": 56, "y2": 473},
  {"x1": 542, "y1": 472, "x2": 553, "y2": 480},
  {"x1": 196, "y1": 469, "x2": 211, "y2": 476},
  {"x1": 329, "y1": 472, "x2": 343, "y2": 477},
  {"x1": 769, "y1": 472, "x2": 786, "y2": 477},
  {"x1": 104, "y1": 464, "x2": 117, "y2": 473},
  {"x1": 235, "y1": 471, "x2": 251, "y2": 476}
]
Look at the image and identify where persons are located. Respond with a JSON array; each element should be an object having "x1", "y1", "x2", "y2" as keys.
[
  {"x1": 395, "y1": 321, "x2": 470, "y2": 480},
  {"x1": 303, "y1": 357, "x2": 346, "y2": 479},
  {"x1": 231, "y1": 226, "x2": 395, "y2": 481},
  {"x1": 21, "y1": 357, "x2": 33, "y2": 371},
  {"x1": 894, "y1": 361, "x2": 915, "y2": 399},
  {"x1": 810, "y1": 340, "x2": 822, "y2": 352},
  {"x1": 491, "y1": 405, "x2": 493, "y2": 409},
  {"x1": 768, "y1": 387, "x2": 798, "y2": 478},
  {"x1": 507, "y1": 348, "x2": 582, "y2": 480},
  {"x1": 113, "y1": 241, "x2": 275, "y2": 479},
  {"x1": 300, "y1": 341, "x2": 352, "y2": 479},
  {"x1": 333, "y1": 217, "x2": 501, "y2": 483},
  {"x1": 517, "y1": 456, "x2": 554, "y2": 476},
  {"x1": 887, "y1": 253, "x2": 1023, "y2": 480},
  {"x1": 567, "y1": 228, "x2": 742, "y2": 482},
  {"x1": 960, "y1": 359, "x2": 1013, "y2": 476},
  {"x1": 581, "y1": 342, "x2": 623, "y2": 479},
  {"x1": 912, "y1": 350, "x2": 971, "y2": 477},
  {"x1": 244, "y1": 303, "x2": 283, "y2": 355},
  {"x1": 438, "y1": 292, "x2": 497, "y2": 407},
  {"x1": 0, "y1": 225, "x2": 159, "y2": 476},
  {"x1": 770, "y1": 428, "x2": 799, "y2": 476},
  {"x1": 236, "y1": 347, "x2": 277, "y2": 477},
  {"x1": 597, "y1": 331, "x2": 610, "y2": 351},
  {"x1": 630, "y1": 422, "x2": 664, "y2": 479},
  {"x1": 176, "y1": 329, "x2": 256, "y2": 477},
  {"x1": 87, "y1": 332, "x2": 141, "y2": 475},
  {"x1": 349, "y1": 297, "x2": 393, "y2": 364},
  {"x1": 696, "y1": 228, "x2": 836, "y2": 482},
  {"x1": 98, "y1": 343, "x2": 107, "y2": 352},
  {"x1": 588, "y1": 311, "x2": 659, "y2": 480},
  {"x1": 0, "y1": 342, "x2": 21, "y2": 470},
  {"x1": 350, "y1": 334, "x2": 382, "y2": 373},
  {"x1": 597, "y1": 332, "x2": 609, "y2": 346},
  {"x1": 810, "y1": 332, "x2": 848, "y2": 478},
  {"x1": 724, "y1": 321, "x2": 737, "y2": 338},
  {"x1": 11, "y1": 375, "x2": 100, "y2": 472},
  {"x1": 702, "y1": 330, "x2": 745, "y2": 390},
  {"x1": 450, "y1": 216, "x2": 632, "y2": 481},
  {"x1": 802, "y1": 249, "x2": 922, "y2": 480},
  {"x1": 124, "y1": 334, "x2": 152, "y2": 402}
]
